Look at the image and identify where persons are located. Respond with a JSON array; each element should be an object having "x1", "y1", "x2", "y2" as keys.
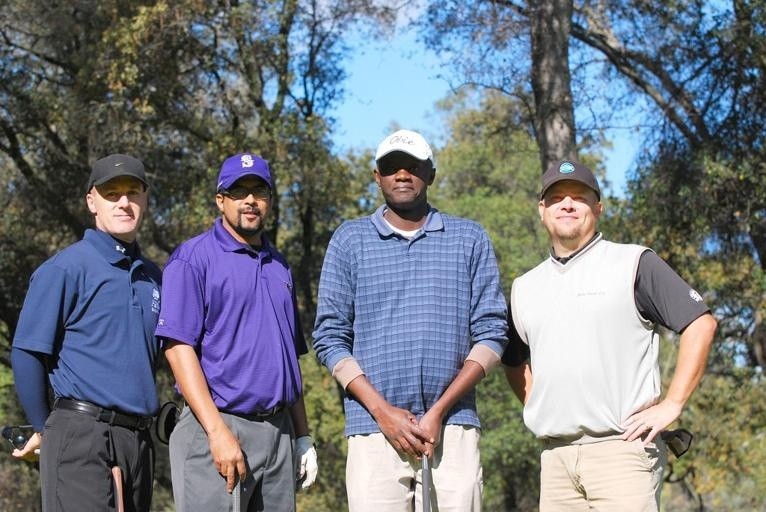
[
  {"x1": 312, "y1": 128, "x2": 510, "y2": 512},
  {"x1": 10, "y1": 153, "x2": 162, "y2": 512},
  {"x1": 501, "y1": 161, "x2": 718, "y2": 512},
  {"x1": 154, "y1": 152, "x2": 318, "y2": 512}
]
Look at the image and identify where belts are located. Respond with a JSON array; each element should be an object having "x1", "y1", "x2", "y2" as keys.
[
  {"x1": 56, "y1": 397, "x2": 153, "y2": 430},
  {"x1": 232, "y1": 405, "x2": 282, "y2": 421}
]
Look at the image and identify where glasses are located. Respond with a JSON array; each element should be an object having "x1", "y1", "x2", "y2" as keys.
[
  {"x1": 222, "y1": 186, "x2": 271, "y2": 200},
  {"x1": 2, "y1": 425, "x2": 34, "y2": 450}
]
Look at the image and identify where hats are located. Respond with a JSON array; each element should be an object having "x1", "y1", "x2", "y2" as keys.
[
  {"x1": 376, "y1": 130, "x2": 436, "y2": 167},
  {"x1": 216, "y1": 152, "x2": 272, "y2": 192},
  {"x1": 86, "y1": 154, "x2": 149, "y2": 192},
  {"x1": 540, "y1": 161, "x2": 602, "y2": 200}
]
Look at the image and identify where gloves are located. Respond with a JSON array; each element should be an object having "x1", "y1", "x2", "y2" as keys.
[{"x1": 292, "y1": 436, "x2": 319, "y2": 491}]
